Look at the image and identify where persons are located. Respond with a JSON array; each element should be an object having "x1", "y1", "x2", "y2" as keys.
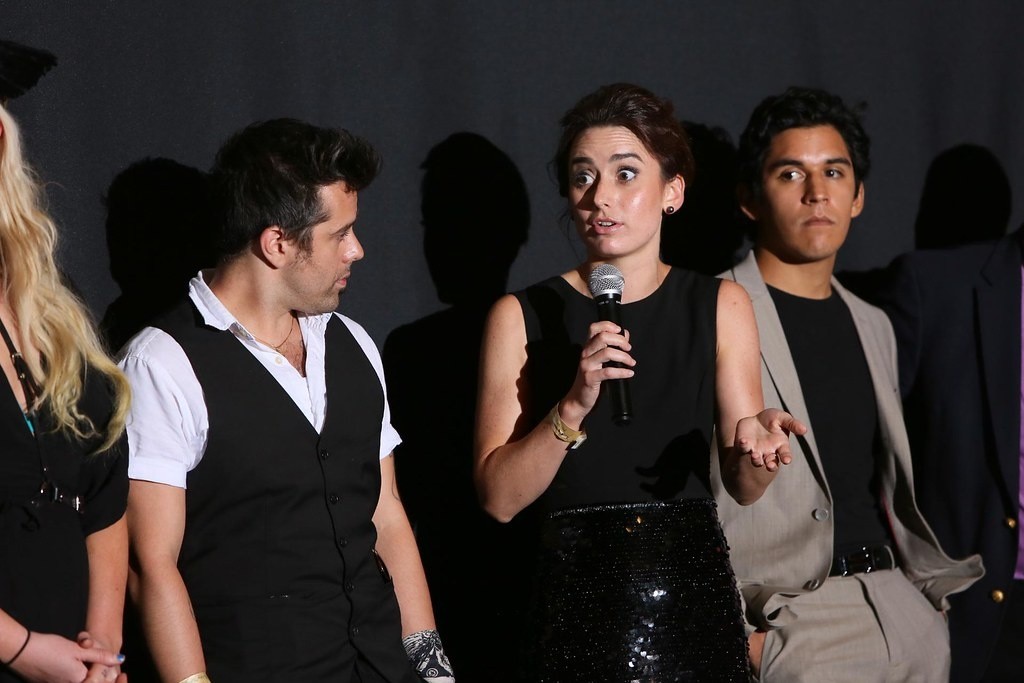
[
  {"x1": 0, "y1": 103, "x2": 134, "y2": 683},
  {"x1": 108, "y1": 119, "x2": 454, "y2": 682},
  {"x1": 876, "y1": 222, "x2": 1024, "y2": 683},
  {"x1": 711, "y1": 88, "x2": 984, "y2": 682},
  {"x1": 470, "y1": 82, "x2": 808, "y2": 683}
]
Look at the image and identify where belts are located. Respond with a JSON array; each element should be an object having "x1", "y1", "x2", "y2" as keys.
[{"x1": 830, "y1": 547, "x2": 898, "y2": 576}]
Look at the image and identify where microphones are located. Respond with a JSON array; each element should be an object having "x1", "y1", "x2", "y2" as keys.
[{"x1": 587, "y1": 264, "x2": 633, "y2": 427}]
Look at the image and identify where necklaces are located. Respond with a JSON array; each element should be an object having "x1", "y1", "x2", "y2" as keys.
[{"x1": 259, "y1": 315, "x2": 294, "y2": 353}]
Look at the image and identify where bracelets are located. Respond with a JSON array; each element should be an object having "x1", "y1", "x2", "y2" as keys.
[
  {"x1": 548, "y1": 401, "x2": 587, "y2": 450},
  {"x1": 4, "y1": 627, "x2": 31, "y2": 666},
  {"x1": 181, "y1": 672, "x2": 210, "y2": 683}
]
